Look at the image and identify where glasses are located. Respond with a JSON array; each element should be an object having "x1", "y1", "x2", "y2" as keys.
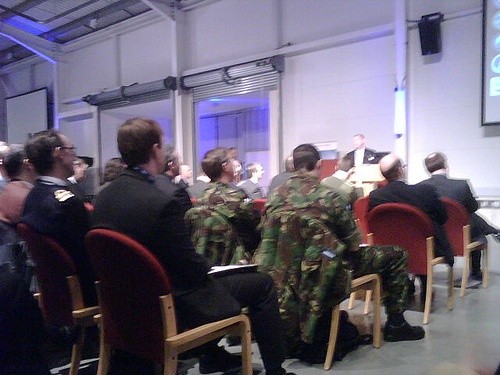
[
  {"x1": 61, "y1": 145, "x2": 77, "y2": 152},
  {"x1": 74, "y1": 162, "x2": 89, "y2": 168}
]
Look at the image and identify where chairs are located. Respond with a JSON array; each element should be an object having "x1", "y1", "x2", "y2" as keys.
[{"x1": 16, "y1": 199, "x2": 489, "y2": 375}]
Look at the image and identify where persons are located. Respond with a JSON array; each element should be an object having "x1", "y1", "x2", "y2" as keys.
[
  {"x1": 185, "y1": 167, "x2": 211, "y2": 198},
  {"x1": 229, "y1": 159, "x2": 246, "y2": 192},
  {"x1": 160, "y1": 144, "x2": 179, "y2": 180},
  {"x1": 347, "y1": 134, "x2": 376, "y2": 164},
  {"x1": 421, "y1": 152, "x2": 500, "y2": 281},
  {"x1": 264, "y1": 144, "x2": 425, "y2": 342},
  {"x1": 239, "y1": 162, "x2": 265, "y2": 197},
  {"x1": 267, "y1": 155, "x2": 295, "y2": 196},
  {"x1": 368, "y1": 154, "x2": 454, "y2": 302},
  {"x1": 91, "y1": 117, "x2": 295, "y2": 375},
  {"x1": 0, "y1": 129, "x2": 99, "y2": 307},
  {"x1": 92, "y1": 158, "x2": 127, "y2": 206},
  {"x1": 199, "y1": 148, "x2": 261, "y2": 253},
  {"x1": 319, "y1": 155, "x2": 357, "y2": 204},
  {"x1": 178, "y1": 165, "x2": 193, "y2": 188}
]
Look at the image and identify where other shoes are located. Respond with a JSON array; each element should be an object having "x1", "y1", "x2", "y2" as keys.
[
  {"x1": 383, "y1": 320, "x2": 425, "y2": 342},
  {"x1": 198, "y1": 345, "x2": 243, "y2": 375},
  {"x1": 470, "y1": 268, "x2": 482, "y2": 281}
]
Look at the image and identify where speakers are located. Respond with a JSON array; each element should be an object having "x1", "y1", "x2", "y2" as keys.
[{"x1": 418, "y1": 18, "x2": 441, "y2": 55}]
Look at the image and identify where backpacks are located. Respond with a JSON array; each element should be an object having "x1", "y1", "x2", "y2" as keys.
[{"x1": 299, "y1": 309, "x2": 374, "y2": 364}]
